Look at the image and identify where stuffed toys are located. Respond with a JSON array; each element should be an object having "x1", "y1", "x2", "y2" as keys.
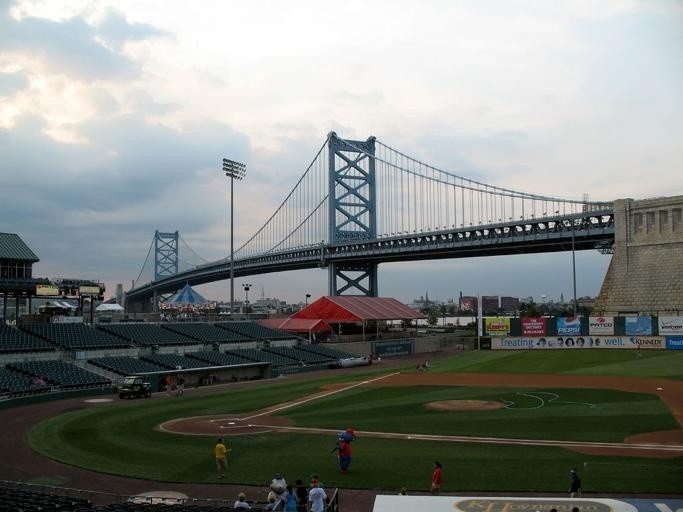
[{"x1": 329, "y1": 427, "x2": 354, "y2": 474}]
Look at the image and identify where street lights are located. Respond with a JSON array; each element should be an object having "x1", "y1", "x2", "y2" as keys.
[
  {"x1": 306, "y1": 294, "x2": 311, "y2": 308},
  {"x1": 223, "y1": 158, "x2": 246, "y2": 313},
  {"x1": 243, "y1": 284, "x2": 252, "y2": 320}
]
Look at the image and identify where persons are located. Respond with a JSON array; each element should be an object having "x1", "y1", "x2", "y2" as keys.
[
  {"x1": 233, "y1": 492, "x2": 250, "y2": 509},
  {"x1": 176, "y1": 373, "x2": 184, "y2": 397},
  {"x1": 430, "y1": 460, "x2": 442, "y2": 496},
  {"x1": 397, "y1": 486, "x2": 408, "y2": 496},
  {"x1": 422, "y1": 358, "x2": 430, "y2": 367},
  {"x1": 297, "y1": 356, "x2": 305, "y2": 366},
  {"x1": 415, "y1": 362, "x2": 421, "y2": 371},
  {"x1": 36, "y1": 374, "x2": 44, "y2": 385},
  {"x1": 214, "y1": 437, "x2": 231, "y2": 479},
  {"x1": 263, "y1": 472, "x2": 328, "y2": 511},
  {"x1": 567, "y1": 467, "x2": 581, "y2": 497},
  {"x1": 175, "y1": 363, "x2": 182, "y2": 369},
  {"x1": 535, "y1": 338, "x2": 600, "y2": 348},
  {"x1": 165, "y1": 374, "x2": 172, "y2": 393},
  {"x1": 634, "y1": 345, "x2": 641, "y2": 359}
]
